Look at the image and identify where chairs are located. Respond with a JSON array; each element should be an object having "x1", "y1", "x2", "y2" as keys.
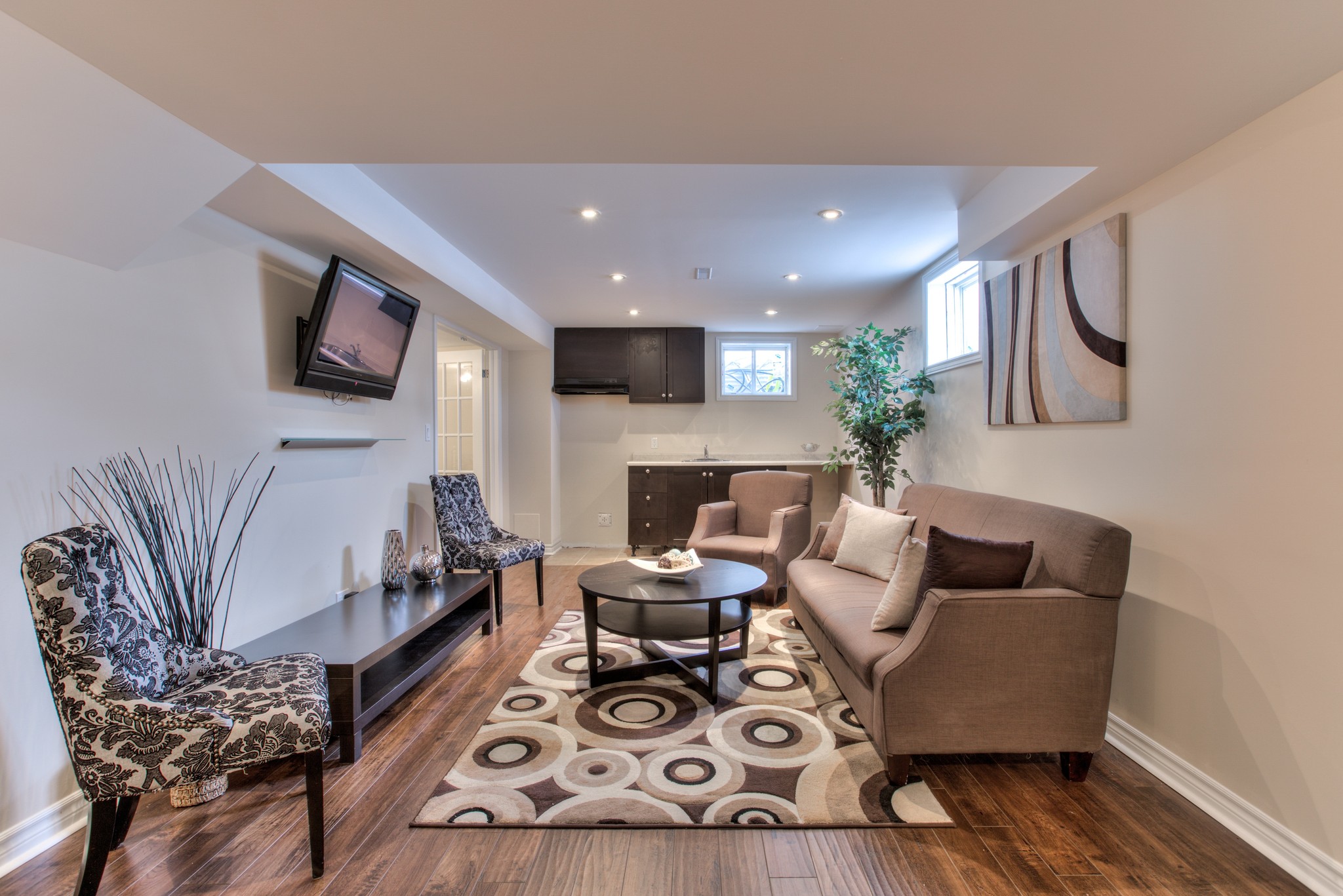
[
  {"x1": 22, "y1": 524, "x2": 333, "y2": 896},
  {"x1": 429, "y1": 473, "x2": 545, "y2": 625},
  {"x1": 685, "y1": 471, "x2": 813, "y2": 607}
]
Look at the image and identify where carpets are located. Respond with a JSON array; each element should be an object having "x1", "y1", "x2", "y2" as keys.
[{"x1": 408, "y1": 610, "x2": 957, "y2": 828}]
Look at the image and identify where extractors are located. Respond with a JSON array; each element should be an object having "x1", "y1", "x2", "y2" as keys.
[{"x1": 554, "y1": 378, "x2": 629, "y2": 394}]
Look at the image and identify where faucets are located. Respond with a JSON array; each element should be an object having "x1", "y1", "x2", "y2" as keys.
[{"x1": 704, "y1": 444, "x2": 709, "y2": 459}]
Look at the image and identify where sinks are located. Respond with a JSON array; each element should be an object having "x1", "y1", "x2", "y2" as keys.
[{"x1": 682, "y1": 459, "x2": 733, "y2": 462}]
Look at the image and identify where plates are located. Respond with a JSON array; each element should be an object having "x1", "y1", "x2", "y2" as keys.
[{"x1": 627, "y1": 547, "x2": 704, "y2": 580}]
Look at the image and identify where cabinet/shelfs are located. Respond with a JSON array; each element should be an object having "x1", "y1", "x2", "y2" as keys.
[
  {"x1": 629, "y1": 327, "x2": 705, "y2": 404},
  {"x1": 628, "y1": 465, "x2": 786, "y2": 557}
]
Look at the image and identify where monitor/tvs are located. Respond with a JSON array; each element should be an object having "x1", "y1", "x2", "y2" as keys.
[{"x1": 294, "y1": 254, "x2": 421, "y2": 401}]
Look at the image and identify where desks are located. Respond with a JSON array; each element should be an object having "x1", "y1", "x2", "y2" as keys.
[
  {"x1": 578, "y1": 557, "x2": 767, "y2": 704},
  {"x1": 227, "y1": 573, "x2": 494, "y2": 762}
]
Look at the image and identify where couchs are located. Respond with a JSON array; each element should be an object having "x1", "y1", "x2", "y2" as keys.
[{"x1": 786, "y1": 483, "x2": 1133, "y2": 782}]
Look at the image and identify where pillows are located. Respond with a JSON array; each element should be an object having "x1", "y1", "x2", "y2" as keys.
[
  {"x1": 913, "y1": 526, "x2": 1034, "y2": 621},
  {"x1": 831, "y1": 499, "x2": 917, "y2": 583},
  {"x1": 871, "y1": 535, "x2": 927, "y2": 631},
  {"x1": 817, "y1": 492, "x2": 908, "y2": 560}
]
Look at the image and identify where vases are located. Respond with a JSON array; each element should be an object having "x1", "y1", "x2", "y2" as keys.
[
  {"x1": 409, "y1": 544, "x2": 444, "y2": 583},
  {"x1": 380, "y1": 530, "x2": 407, "y2": 590}
]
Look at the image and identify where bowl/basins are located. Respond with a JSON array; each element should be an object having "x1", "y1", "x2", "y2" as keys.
[{"x1": 801, "y1": 443, "x2": 820, "y2": 452}]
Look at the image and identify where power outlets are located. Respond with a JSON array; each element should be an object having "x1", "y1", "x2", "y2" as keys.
[
  {"x1": 651, "y1": 438, "x2": 658, "y2": 448},
  {"x1": 599, "y1": 513, "x2": 612, "y2": 526}
]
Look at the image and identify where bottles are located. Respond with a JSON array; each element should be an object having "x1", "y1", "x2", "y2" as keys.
[
  {"x1": 381, "y1": 529, "x2": 407, "y2": 590},
  {"x1": 409, "y1": 544, "x2": 444, "y2": 583}
]
